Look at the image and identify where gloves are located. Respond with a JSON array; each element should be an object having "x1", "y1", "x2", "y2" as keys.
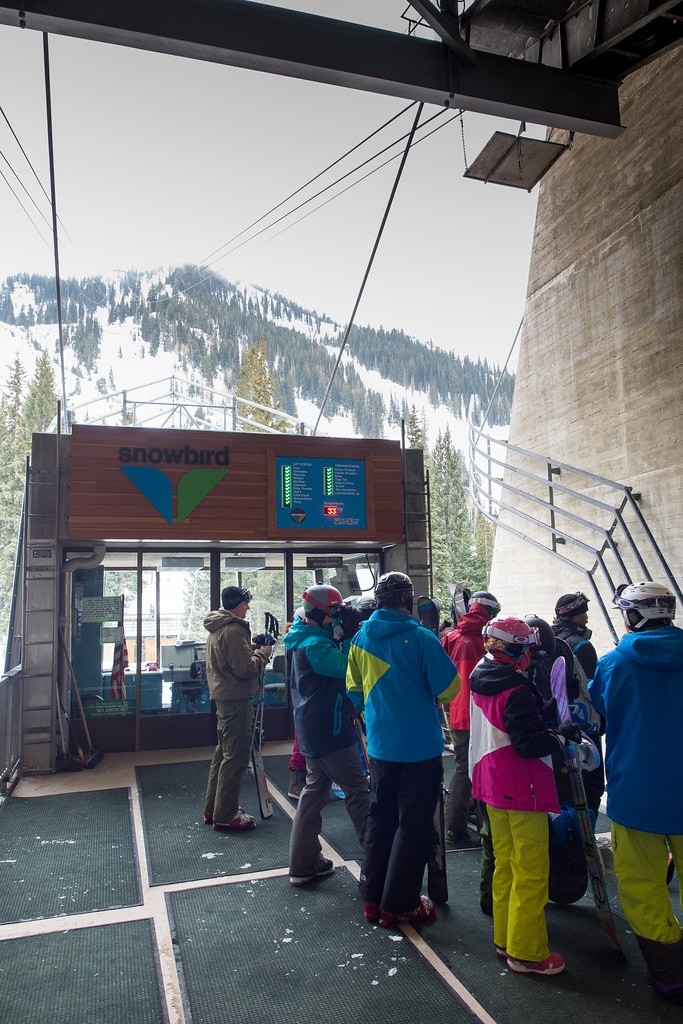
[
  {"x1": 542, "y1": 698, "x2": 557, "y2": 721},
  {"x1": 549, "y1": 719, "x2": 582, "y2": 750}
]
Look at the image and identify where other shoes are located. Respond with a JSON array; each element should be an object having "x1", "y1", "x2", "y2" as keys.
[
  {"x1": 288, "y1": 782, "x2": 305, "y2": 799},
  {"x1": 203, "y1": 806, "x2": 244, "y2": 824},
  {"x1": 212, "y1": 816, "x2": 255, "y2": 830},
  {"x1": 364, "y1": 898, "x2": 380, "y2": 922},
  {"x1": 445, "y1": 831, "x2": 481, "y2": 853},
  {"x1": 378, "y1": 894, "x2": 437, "y2": 927}
]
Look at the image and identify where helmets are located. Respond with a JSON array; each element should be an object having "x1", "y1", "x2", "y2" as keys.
[
  {"x1": 482, "y1": 618, "x2": 535, "y2": 663},
  {"x1": 522, "y1": 619, "x2": 556, "y2": 661},
  {"x1": 374, "y1": 571, "x2": 414, "y2": 605},
  {"x1": 469, "y1": 591, "x2": 501, "y2": 620},
  {"x1": 302, "y1": 583, "x2": 342, "y2": 619},
  {"x1": 613, "y1": 583, "x2": 676, "y2": 631}
]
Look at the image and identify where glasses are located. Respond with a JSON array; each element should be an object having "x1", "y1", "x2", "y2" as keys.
[
  {"x1": 327, "y1": 607, "x2": 344, "y2": 619},
  {"x1": 612, "y1": 584, "x2": 629, "y2": 605},
  {"x1": 576, "y1": 592, "x2": 590, "y2": 607},
  {"x1": 531, "y1": 627, "x2": 543, "y2": 646}
]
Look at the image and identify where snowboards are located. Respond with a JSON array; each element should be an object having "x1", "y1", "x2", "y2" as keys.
[{"x1": 549, "y1": 656, "x2": 632, "y2": 961}]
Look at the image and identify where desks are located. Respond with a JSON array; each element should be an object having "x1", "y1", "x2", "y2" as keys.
[{"x1": 252, "y1": 669, "x2": 288, "y2": 706}]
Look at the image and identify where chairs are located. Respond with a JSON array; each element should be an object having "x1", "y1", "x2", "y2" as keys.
[{"x1": 271, "y1": 654, "x2": 287, "y2": 672}]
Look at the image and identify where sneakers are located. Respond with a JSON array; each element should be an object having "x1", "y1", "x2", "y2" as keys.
[
  {"x1": 645, "y1": 966, "x2": 683, "y2": 1007},
  {"x1": 496, "y1": 945, "x2": 508, "y2": 958},
  {"x1": 289, "y1": 858, "x2": 333, "y2": 885},
  {"x1": 507, "y1": 951, "x2": 565, "y2": 974}
]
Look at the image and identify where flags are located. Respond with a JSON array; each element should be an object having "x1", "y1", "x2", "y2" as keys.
[{"x1": 109, "y1": 606, "x2": 128, "y2": 701}]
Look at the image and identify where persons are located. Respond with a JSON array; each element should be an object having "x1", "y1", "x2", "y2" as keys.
[
  {"x1": 467, "y1": 618, "x2": 581, "y2": 975},
  {"x1": 586, "y1": 580, "x2": 683, "y2": 1003},
  {"x1": 345, "y1": 571, "x2": 462, "y2": 927},
  {"x1": 203, "y1": 586, "x2": 272, "y2": 832},
  {"x1": 283, "y1": 585, "x2": 370, "y2": 885},
  {"x1": 440, "y1": 592, "x2": 605, "y2": 915},
  {"x1": 287, "y1": 598, "x2": 379, "y2": 800}
]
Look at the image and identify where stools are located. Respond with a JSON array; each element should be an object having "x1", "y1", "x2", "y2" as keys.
[
  {"x1": 263, "y1": 684, "x2": 286, "y2": 704},
  {"x1": 178, "y1": 686, "x2": 201, "y2": 713}
]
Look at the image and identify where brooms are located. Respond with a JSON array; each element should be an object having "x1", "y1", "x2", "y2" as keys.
[{"x1": 58, "y1": 622, "x2": 104, "y2": 770}]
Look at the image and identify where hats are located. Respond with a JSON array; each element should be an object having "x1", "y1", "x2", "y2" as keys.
[
  {"x1": 222, "y1": 586, "x2": 250, "y2": 609},
  {"x1": 554, "y1": 594, "x2": 588, "y2": 619}
]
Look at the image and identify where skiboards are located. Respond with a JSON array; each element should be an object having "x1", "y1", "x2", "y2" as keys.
[
  {"x1": 251, "y1": 704, "x2": 274, "y2": 820},
  {"x1": 446, "y1": 580, "x2": 467, "y2": 621},
  {"x1": 330, "y1": 712, "x2": 371, "y2": 800},
  {"x1": 417, "y1": 595, "x2": 447, "y2": 907}
]
